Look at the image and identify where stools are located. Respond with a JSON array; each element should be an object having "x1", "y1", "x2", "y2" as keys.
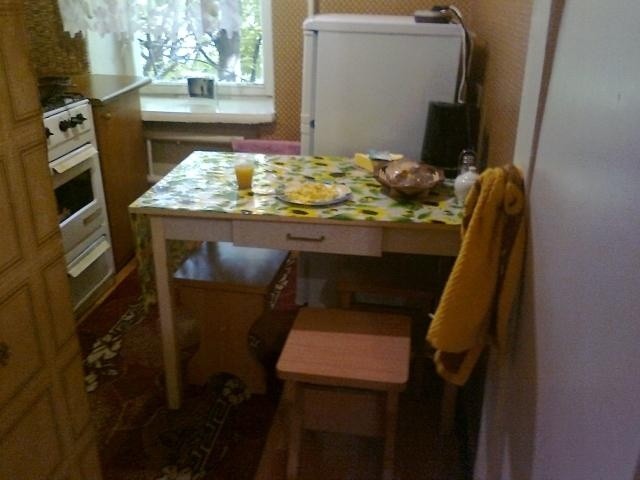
[
  {"x1": 174, "y1": 238, "x2": 291, "y2": 394},
  {"x1": 275, "y1": 306, "x2": 414, "y2": 479}
]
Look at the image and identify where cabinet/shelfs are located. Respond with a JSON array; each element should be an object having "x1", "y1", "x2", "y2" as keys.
[{"x1": 63, "y1": 74, "x2": 153, "y2": 274}]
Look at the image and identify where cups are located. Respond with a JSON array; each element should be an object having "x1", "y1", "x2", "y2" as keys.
[
  {"x1": 235, "y1": 162, "x2": 254, "y2": 188},
  {"x1": 453, "y1": 167, "x2": 480, "y2": 206}
]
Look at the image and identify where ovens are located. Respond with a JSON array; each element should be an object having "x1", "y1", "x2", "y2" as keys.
[{"x1": 48, "y1": 143, "x2": 104, "y2": 256}]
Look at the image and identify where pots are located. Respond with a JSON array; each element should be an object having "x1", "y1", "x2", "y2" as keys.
[{"x1": 37, "y1": 73, "x2": 77, "y2": 99}]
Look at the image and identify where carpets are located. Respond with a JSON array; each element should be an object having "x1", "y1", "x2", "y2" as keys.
[{"x1": 75, "y1": 212, "x2": 286, "y2": 479}]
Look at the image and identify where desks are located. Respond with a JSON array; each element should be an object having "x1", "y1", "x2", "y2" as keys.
[{"x1": 127, "y1": 150, "x2": 468, "y2": 408}]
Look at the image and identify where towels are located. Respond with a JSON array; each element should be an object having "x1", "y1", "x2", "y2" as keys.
[{"x1": 425, "y1": 163, "x2": 529, "y2": 387}]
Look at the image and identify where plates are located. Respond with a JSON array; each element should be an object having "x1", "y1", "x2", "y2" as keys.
[{"x1": 274, "y1": 179, "x2": 353, "y2": 206}]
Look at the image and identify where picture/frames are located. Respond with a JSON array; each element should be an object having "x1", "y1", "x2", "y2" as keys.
[{"x1": 187, "y1": 77, "x2": 216, "y2": 102}]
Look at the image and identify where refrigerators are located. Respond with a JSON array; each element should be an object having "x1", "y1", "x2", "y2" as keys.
[{"x1": 300, "y1": 13, "x2": 465, "y2": 159}]
